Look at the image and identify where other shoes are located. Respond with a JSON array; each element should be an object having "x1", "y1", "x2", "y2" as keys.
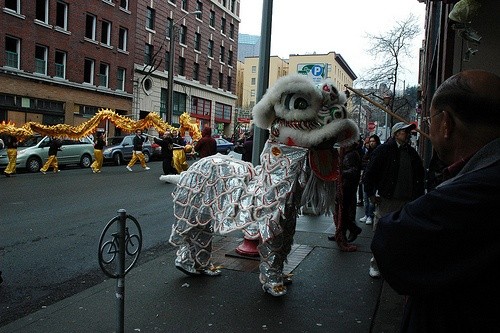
[
  {"x1": 126, "y1": 166, "x2": 132, "y2": 171},
  {"x1": 145, "y1": 167, "x2": 151, "y2": 170},
  {"x1": 328, "y1": 226, "x2": 362, "y2": 242}
]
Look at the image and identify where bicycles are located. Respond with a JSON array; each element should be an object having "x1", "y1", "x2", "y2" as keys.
[{"x1": 99, "y1": 227, "x2": 140, "y2": 264}]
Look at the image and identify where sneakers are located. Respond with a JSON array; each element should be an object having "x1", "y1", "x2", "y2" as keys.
[
  {"x1": 359, "y1": 215, "x2": 372, "y2": 225},
  {"x1": 369, "y1": 258, "x2": 380, "y2": 278}
]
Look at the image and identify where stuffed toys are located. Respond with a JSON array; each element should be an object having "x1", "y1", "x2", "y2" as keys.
[{"x1": 155, "y1": 71, "x2": 361, "y2": 298}]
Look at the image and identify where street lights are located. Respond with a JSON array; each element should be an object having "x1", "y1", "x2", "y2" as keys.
[
  {"x1": 165, "y1": 10, "x2": 202, "y2": 126},
  {"x1": 383, "y1": 96, "x2": 390, "y2": 142}
]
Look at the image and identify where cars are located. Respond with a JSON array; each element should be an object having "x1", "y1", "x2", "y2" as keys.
[
  {"x1": 152, "y1": 138, "x2": 195, "y2": 159},
  {"x1": 214, "y1": 138, "x2": 236, "y2": 154}
]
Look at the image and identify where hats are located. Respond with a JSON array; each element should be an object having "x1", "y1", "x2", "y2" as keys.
[{"x1": 392, "y1": 121, "x2": 417, "y2": 135}]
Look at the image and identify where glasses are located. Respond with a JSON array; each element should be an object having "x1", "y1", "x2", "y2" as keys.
[{"x1": 423, "y1": 111, "x2": 442, "y2": 129}]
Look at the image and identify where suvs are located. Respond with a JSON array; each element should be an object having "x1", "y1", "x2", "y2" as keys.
[
  {"x1": 100, "y1": 136, "x2": 153, "y2": 164},
  {"x1": 0, "y1": 130, "x2": 95, "y2": 174}
]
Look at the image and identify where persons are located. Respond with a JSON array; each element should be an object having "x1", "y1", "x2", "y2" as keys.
[
  {"x1": 126, "y1": 128, "x2": 151, "y2": 172},
  {"x1": 194, "y1": 126, "x2": 219, "y2": 159},
  {"x1": 90, "y1": 129, "x2": 108, "y2": 175},
  {"x1": 325, "y1": 129, "x2": 402, "y2": 243},
  {"x1": 369, "y1": 70, "x2": 499, "y2": 333},
  {"x1": 3, "y1": 134, "x2": 20, "y2": 178},
  {"x1": 364, "y1": 121, "x2": 427, "y2": 278},
  {"x1": 40, "y1": 136, "x2": 63, "y2": 175},
  {"x1": 152, "y1": 125, "x2": 190, "y2": 184},
  {"x1": 234, "y1": 124, "x2": 257, "y2": 162}
]
